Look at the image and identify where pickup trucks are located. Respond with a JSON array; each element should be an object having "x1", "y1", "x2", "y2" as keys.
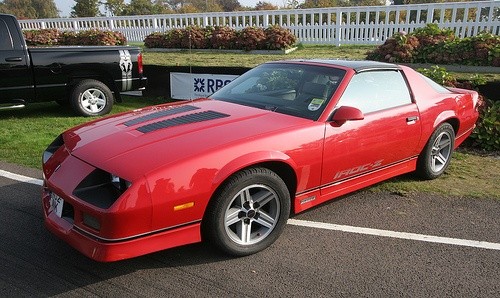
[{"x1": 0, "y1": 15, "x2": 146, "y2": 116}]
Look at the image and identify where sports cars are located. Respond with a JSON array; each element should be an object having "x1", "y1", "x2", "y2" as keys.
[{"x1": 41, "y1": 58, "x2": 480, "y2": 264}]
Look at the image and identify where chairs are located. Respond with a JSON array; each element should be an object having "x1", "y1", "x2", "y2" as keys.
[{"x1": 290, "y1": 81, "x2": 327, "y2": 110}]
